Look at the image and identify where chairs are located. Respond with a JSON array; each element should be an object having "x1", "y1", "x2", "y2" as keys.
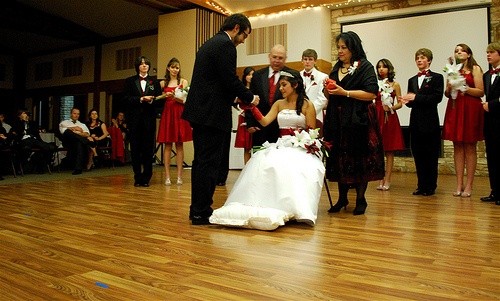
[{"x1": 0, "y1": 129, "x2": 116, "y2": 179}]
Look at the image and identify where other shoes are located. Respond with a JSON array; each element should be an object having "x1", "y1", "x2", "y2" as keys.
[{"x1": 72, "y1": 141, "x2": 100, "y2": 175}]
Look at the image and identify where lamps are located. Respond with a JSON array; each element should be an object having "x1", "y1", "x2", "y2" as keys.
[{"x1": 204, "y1": 0, "x2": 379, "y2": 22}]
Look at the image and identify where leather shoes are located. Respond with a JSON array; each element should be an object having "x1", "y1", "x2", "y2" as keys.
[
  {"x1": 134, "y1": 176, "x2": 150, "y2": 187},
  {"x1": 412, "y1": 187, "x2": 436, "y2": 195},
  {"x1": 479, "y1": 191, "x2": 500, "y2": 205},
  {"x1": 188, "y1": 205, "x2": 218, "y2": 225}
]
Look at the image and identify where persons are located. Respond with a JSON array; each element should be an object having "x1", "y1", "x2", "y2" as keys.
[
  {"x1": 121, "y1": 56, "x2": 166, "y2": 188},
  {"x1": 0, "y1": 107, "x2": 130, "y2": 181},
  {"x1": 234, "y1": 66, "x2": 259, "y2": 166},
  {"x1": 298, "y1": 49, "x2": 330, "y2": 135},
  {"x1": 323, "y1": 31, "x2": 386, "y2": 216},
  {"x1": 372, "y1": 59, "x2": 401, "y2": 191},
  {"x1": 154, "y1": 58, "x2": 194, "y2": 186},
  {"x1": 444, "y1": 44, "x2": 486, "y2": 197},
  {"x1": 210, "y1": 70, "x2": 327, "y2": 230},
  {"x1": 480, "y1": 43, "x2": 500, "y2": 206},
  {"x1": 398, "y1": 48, "x2": 444, "y2": 197},
  {"x1": 245, "y1": 44, "x2": 308, "y2": 156},
  {"x1": 180, "y1": 12, "x2": 260, "y2": 226}
]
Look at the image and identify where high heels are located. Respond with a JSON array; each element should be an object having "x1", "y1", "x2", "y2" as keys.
[
  {"x1": 328, "y1": 197, "x2": 367, "y2": 215},
  {"x1": 376, "y1": 179, "x2": 392, "y2": 190},
  {"x1": 164, "y1": 175, "x2": 183, "y2": 186},
  {"x1": 453, "y1": 185, "x2": 473, "y2": 197}
]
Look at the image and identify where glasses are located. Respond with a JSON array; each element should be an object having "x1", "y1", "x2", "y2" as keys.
[
  {"x1": 304, "y1": 58, "x2": 314, "y2": 62},
  {"x1": 243, "y1": 31, "x2": 248, "y2": 38},
  {"x1": 270, "y1": 54, "x2": 285, "y2": 60}
]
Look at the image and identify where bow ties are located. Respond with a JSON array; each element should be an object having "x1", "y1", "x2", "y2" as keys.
[
  {"x1": 303, "y1": 71, "x2": 312, "y2": 77},
  {"x1": 138, "y1": 76, "x2": 147, "y2": 81},
  {"x1": 489, "y1": 67, "x2": 500, "y2": 75},
  {"x1": 418, "y1": 70, "x2": 426, "y2": 77}
]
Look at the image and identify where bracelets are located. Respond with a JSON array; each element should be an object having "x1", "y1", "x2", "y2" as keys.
[{"x1": 347, "y1": 90, "x2": 350, "y2": 98}]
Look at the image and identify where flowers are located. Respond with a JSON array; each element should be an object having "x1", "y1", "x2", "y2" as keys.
[
  {"x1": 275, "y1": 126, "x2": 333, "y2": 158},
  {"x1": 376, "y1": 76, "x2": 396, "y2": 123},
  {"x1": 147, "y1": 80, "x2": 155, "y2": 91},
  {"x1": 153, "y1": 84, "x2": 192, "y2": 104},
  {"x1": 442, "y1": 58, "x2": 468, "y2": 100}
]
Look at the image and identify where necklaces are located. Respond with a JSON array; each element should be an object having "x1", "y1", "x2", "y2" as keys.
[{"x1": 340, "y1": 67, "x2": 351, "y2": 74}]
